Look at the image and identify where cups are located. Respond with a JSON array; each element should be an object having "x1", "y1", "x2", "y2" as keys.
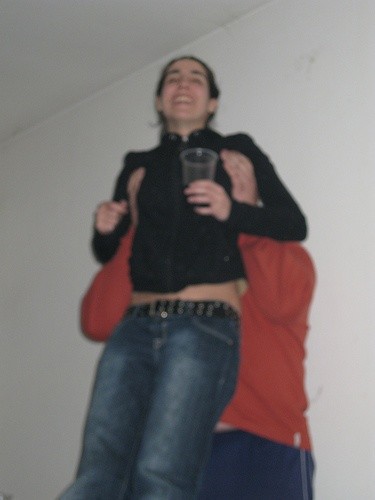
[{"x1": 180, "y1": 149, "x2": 218, "y2": 209}]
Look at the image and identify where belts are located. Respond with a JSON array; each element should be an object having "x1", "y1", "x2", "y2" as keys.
[{"x1": 123, "y1": 299, "x2": 239, "y2": 330}]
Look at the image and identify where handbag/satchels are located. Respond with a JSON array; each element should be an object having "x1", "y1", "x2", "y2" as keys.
[{"x1": 80, "y1": 165, "x2": 144, "y2": 342}]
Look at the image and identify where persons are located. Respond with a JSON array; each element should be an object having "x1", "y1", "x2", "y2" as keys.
[
  {"x1": 54, "y1": 55, "x2": 308, "y2": 500},
  {"x1": 81, "y1": 149, "x2": 316, "y2": 500}
]
